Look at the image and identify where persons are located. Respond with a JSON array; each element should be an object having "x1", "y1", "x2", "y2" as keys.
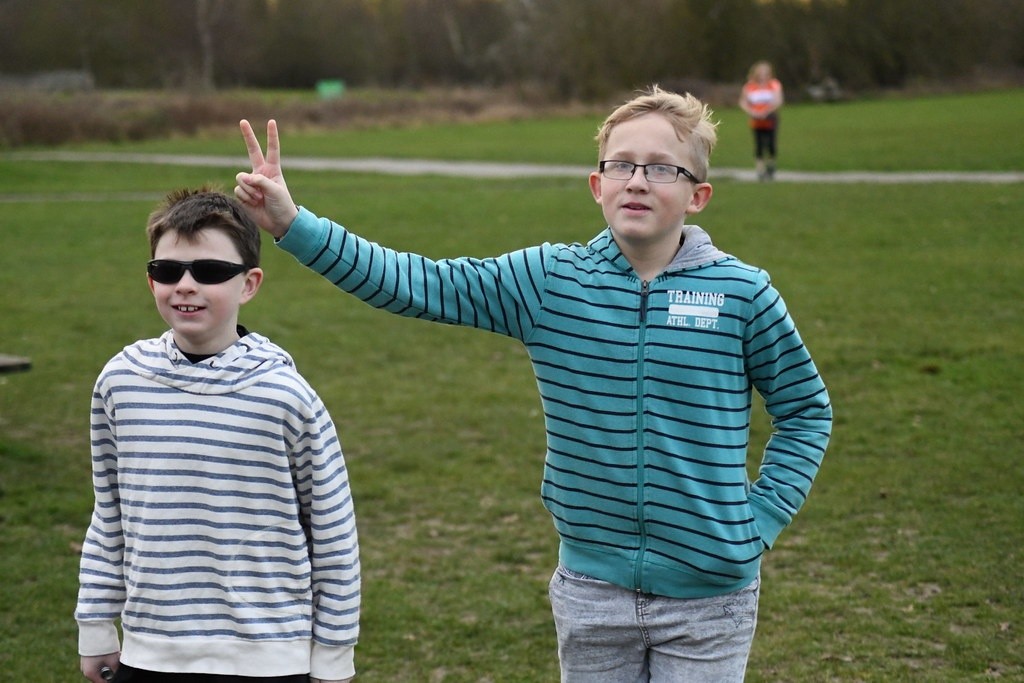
[
  {"x1": 739, "y1": 62, "x2": 783, "y2": 182},
  {"x1": 73, "y1": 186, "x2": 362, "y2": 683},
  {"x1": 235, "y1": 85, "x2": 835, "y2": 683}
]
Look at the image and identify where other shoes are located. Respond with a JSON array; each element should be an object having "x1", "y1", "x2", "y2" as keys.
[{"x1": 755, "y1": 160, "x2": 777, "y2": 178}]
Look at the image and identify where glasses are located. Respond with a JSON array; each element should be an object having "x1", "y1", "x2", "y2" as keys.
[
  {"x1": 599, "y1": 160, "x2": 699, "y2": 183},
  {"x1": 147, "y1": 259, "x2": 250, "y2": 285}
]
